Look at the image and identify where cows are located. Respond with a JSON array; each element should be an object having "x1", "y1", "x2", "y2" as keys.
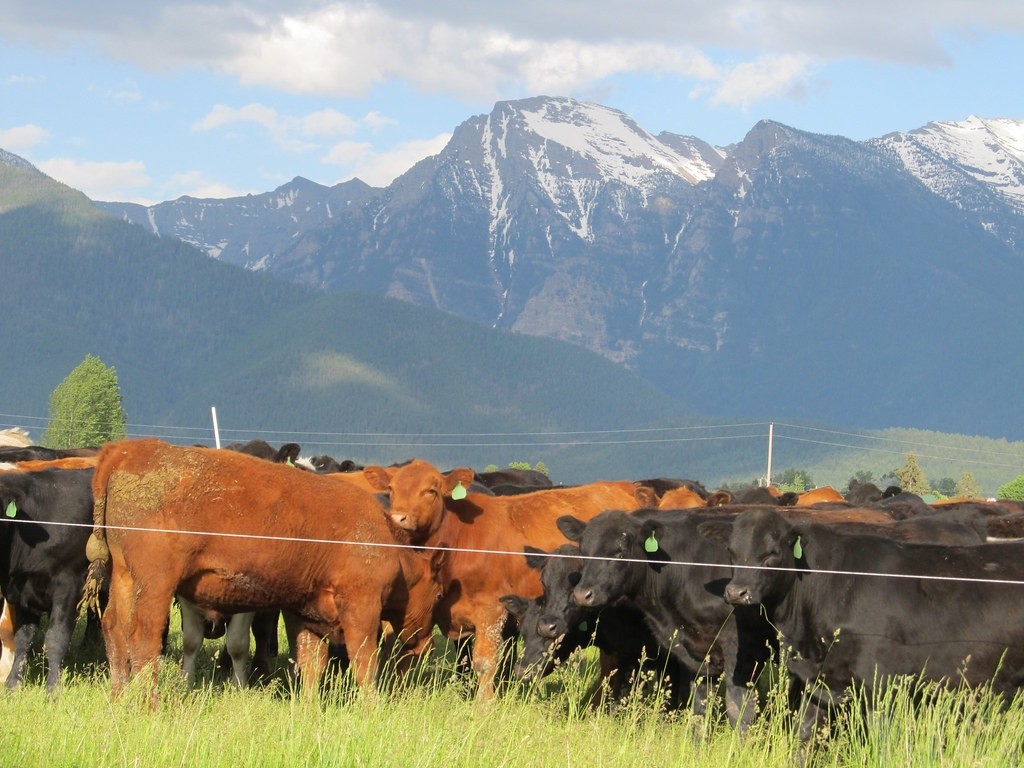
[{"x1": 0, "y1": 431, "x2": 1024, "y2": 768}]
